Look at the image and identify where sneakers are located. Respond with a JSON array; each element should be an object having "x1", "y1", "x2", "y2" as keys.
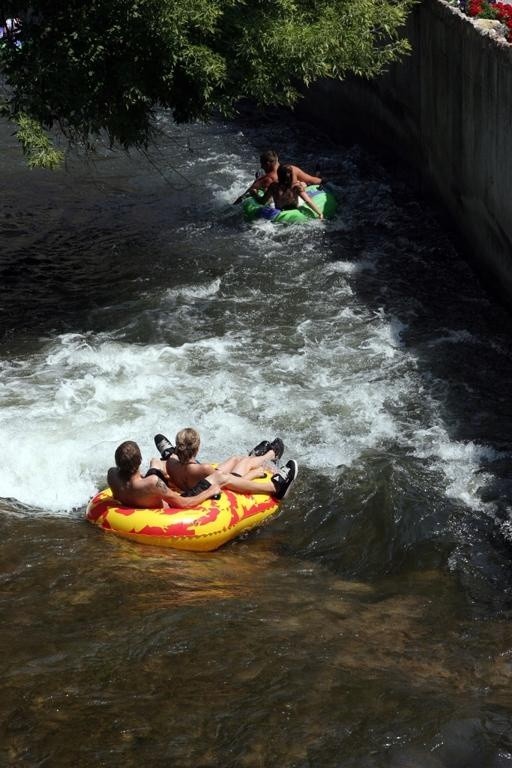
[
  {"x1": 249, "y1": 440, "x2": 270, "y2": 455},
  {"x1": 271, "y1": 458, "x2": 298, "y2": 500},
  {"x1": 154, "y1": 433, "x2": 174, "y2": 458},
  {"x1": 268, "y1": 437, "x2": 284, "y2": 459}
]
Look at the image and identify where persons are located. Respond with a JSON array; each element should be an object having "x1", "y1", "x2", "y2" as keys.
[
  {"x1": 104, "y1": 433, "x2": 299, "y2": 510},
  {"x1": 231, "y1": 148, "x2": 329, "y2": 207},
  {"x1": 247, "y1": 164, "x2": 324, "y2": 220},
  {"x1": 166, "y1": 427, "x2": 287, "y2": 495}
]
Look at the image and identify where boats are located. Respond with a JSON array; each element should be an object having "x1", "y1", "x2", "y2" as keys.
[
  {"x1": 242, "y1": 179, "x2": 339, "y2": 225},
  {"x1": 83, "y1": 463, "x2": 284, "y2": 554}
]
